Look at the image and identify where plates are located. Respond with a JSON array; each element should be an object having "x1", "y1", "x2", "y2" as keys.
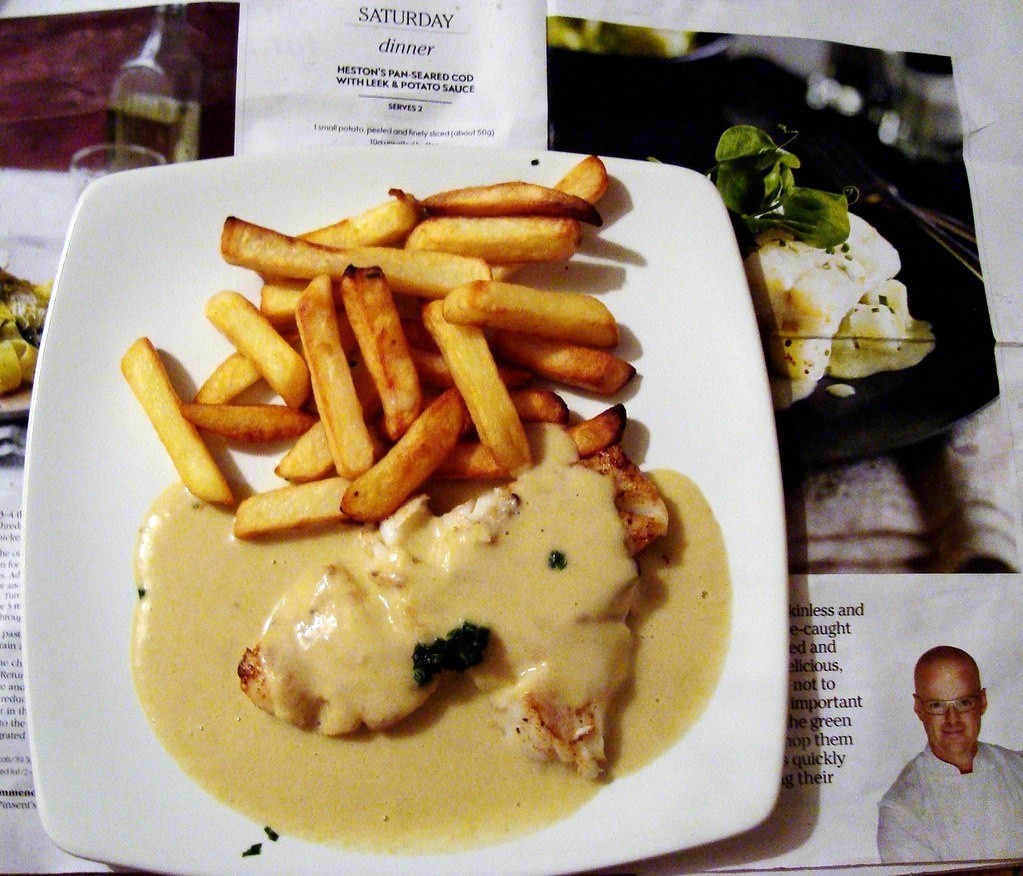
[
  {"x1": 544, "y1": 15, "x2": 999, "y2": 482},
  {"x1": 18, "y1": 144, "x2": 792, "y2": 872}
]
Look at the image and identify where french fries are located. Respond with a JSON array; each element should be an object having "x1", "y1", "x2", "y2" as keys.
[{"x1": 121, "y1": 155, "x2": 638, "y2": 535}]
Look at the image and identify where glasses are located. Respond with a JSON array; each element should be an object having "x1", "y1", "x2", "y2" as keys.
[{"x1": 917, "y1": 691, "x2": 981, "y2": 716}]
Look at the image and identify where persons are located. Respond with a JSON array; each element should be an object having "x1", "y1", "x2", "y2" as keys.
[{"x1": 877, "y1": 650, "x2": 1022, "y2": 864}]
